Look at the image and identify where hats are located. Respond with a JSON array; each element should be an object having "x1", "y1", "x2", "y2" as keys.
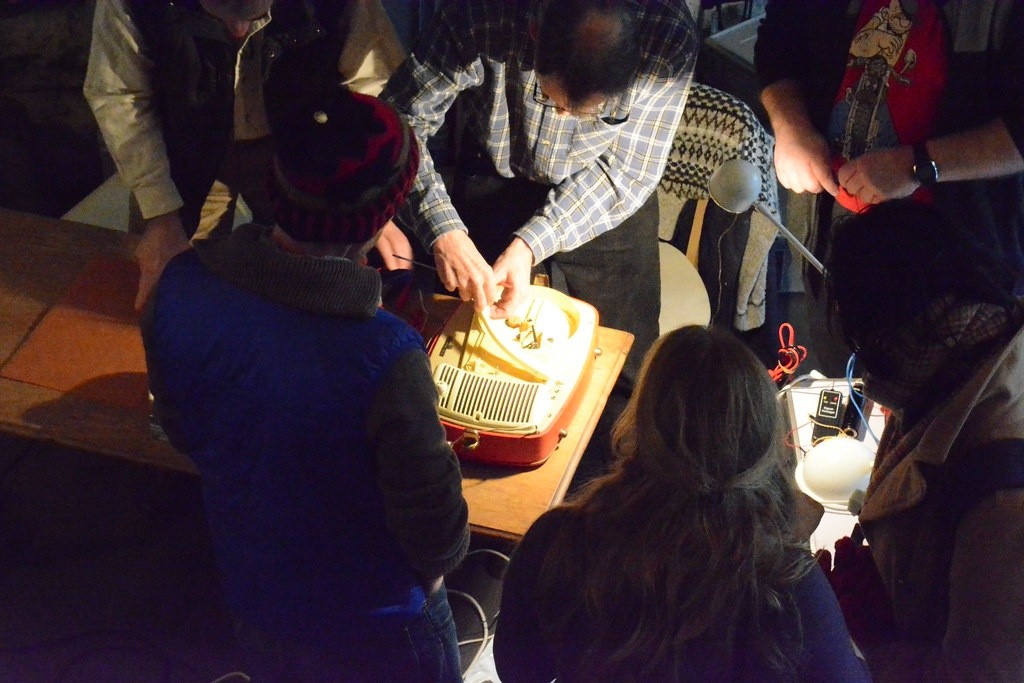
[
  {"x1": 200, "y1": 0, "x2": 272, "y2": 20},
  {"x1": 259, "y1": 91, "x2": 418, "y2": 246}
]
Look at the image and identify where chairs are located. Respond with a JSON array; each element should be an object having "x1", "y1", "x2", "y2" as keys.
[{"x1": 553, "y1": 81, "x2": 784, "y2": 336}]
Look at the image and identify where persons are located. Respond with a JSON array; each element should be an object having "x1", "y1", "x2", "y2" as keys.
[{"x1": 80, "y1": 0, "x2": 1024, "y2": 683}]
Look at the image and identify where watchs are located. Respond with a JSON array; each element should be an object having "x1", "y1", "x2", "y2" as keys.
[{"x1": 911, "y1": 139, "x2": 939, "y2": 187}]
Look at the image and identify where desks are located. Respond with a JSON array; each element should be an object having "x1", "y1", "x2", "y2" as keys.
[
  {"x1": 0, "y1": 206, "x2": 637, "y2": 544},
  {"x1": 786, "y1": 384, "x2": 887, "y2": 563}
]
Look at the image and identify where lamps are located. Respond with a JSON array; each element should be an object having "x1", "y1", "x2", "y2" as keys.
[{"x1": 706, "y1": 157, "x2": 827, "y2": 278}]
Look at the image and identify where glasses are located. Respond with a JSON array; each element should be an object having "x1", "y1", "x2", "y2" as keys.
[{"x1": 533, "y1": 76, "x2": 609, "y2": 123}]
